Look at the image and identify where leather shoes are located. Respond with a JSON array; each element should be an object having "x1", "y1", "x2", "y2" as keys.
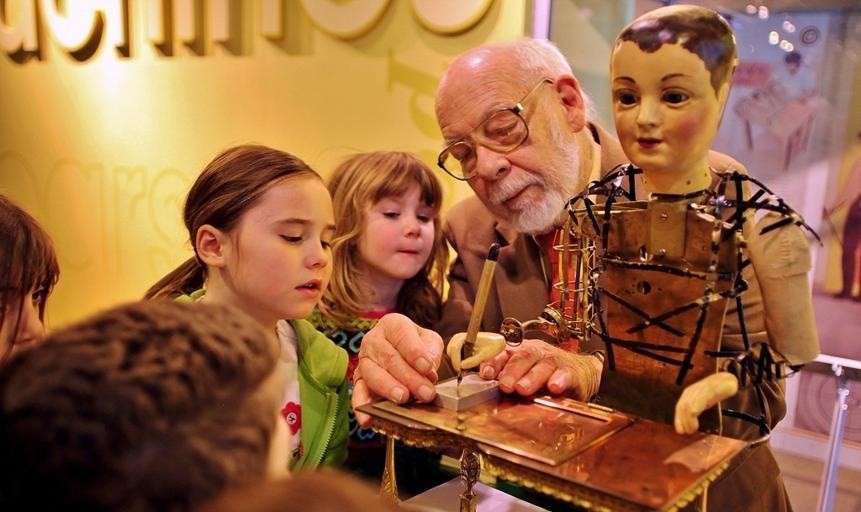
[{"x1": 831, "y1": 290, "x2": 861, "y2": 303}]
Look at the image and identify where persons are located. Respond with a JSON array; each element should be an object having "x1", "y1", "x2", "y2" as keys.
[
  {"x1": 760, "y1": 52, "x2": 817, "y2": 102},
  {"x1": 448, "y1": 4, "x2": 820, "y2": 437},
  {"x1": 209, "y1": 468, "x2": 397, "y2": 510},
  {"x1": 2, "y1": 191, "x2": 58, "y2": 364},
  {"x1": 144, "y1": 146, "x2": 360, "y2": 473},
  {"x1": 1, "y1": 302, "x2": 292, "y2": 512},
  {"x1": 351, "y1": 40, "x2": 799, "y2": 512},
  {"x1": 311, "y1": 150, "x2": 442, "y2": 496}
]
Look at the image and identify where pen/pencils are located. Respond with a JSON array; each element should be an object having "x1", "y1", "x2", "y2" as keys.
[{"x1": 457, "y1": 242, "x2": 500, "y2": 389}]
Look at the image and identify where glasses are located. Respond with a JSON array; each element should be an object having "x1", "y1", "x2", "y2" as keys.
[{"x1": 437, "y1": 77, "x2": 555, "y2": 181}]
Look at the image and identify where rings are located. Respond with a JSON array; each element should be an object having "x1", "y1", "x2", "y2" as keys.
[{"x1": 351, "y1": 370, "x2": 363, "y2": 383}]
